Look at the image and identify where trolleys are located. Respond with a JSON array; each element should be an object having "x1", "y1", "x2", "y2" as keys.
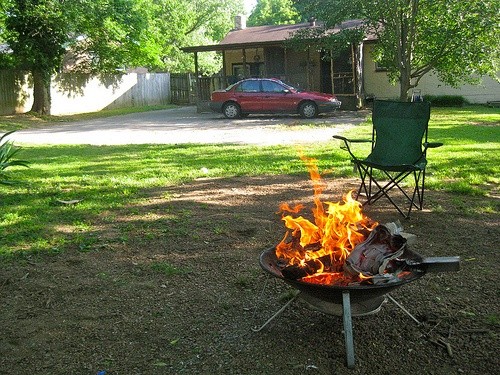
[{"x1": 364, "y1": 94, "x2": 375, "y2": 106}]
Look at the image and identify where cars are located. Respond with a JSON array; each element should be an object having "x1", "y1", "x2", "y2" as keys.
[{"x1": 210, "y1": 78, "x2": 341, "y2": 119}]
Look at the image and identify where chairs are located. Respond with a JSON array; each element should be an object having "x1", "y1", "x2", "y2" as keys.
[{"x1": 334, "y1": 99, "x2": 443, "y2": 219}]
